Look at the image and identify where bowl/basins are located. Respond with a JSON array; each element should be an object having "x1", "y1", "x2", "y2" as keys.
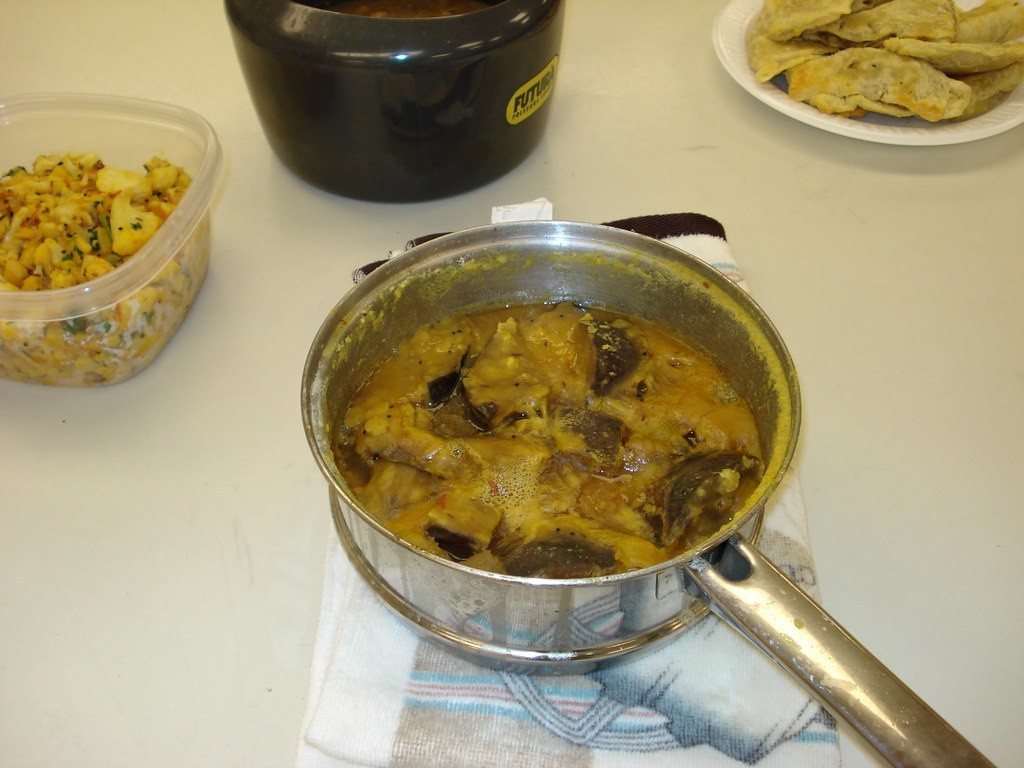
[
  {"x1": 223, "y1": 1, "x2": 565, "y2": 205},
  {"x1": 0, "y1": 93, "x2": 224, "y2": 385}
]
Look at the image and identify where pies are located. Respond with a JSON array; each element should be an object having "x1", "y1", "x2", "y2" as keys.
[{"x1": 744, "y1": 0, "x2": 1024, "y2": 124}]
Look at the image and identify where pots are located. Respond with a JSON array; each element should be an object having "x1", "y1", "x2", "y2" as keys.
[{"x1": 297, "y1": 220, "x2": 1000, "y2": 768}]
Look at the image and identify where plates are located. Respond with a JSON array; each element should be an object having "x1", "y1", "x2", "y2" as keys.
[{"x1": 710, "y1": 0, "x2": 1024, "y2": 146}]
